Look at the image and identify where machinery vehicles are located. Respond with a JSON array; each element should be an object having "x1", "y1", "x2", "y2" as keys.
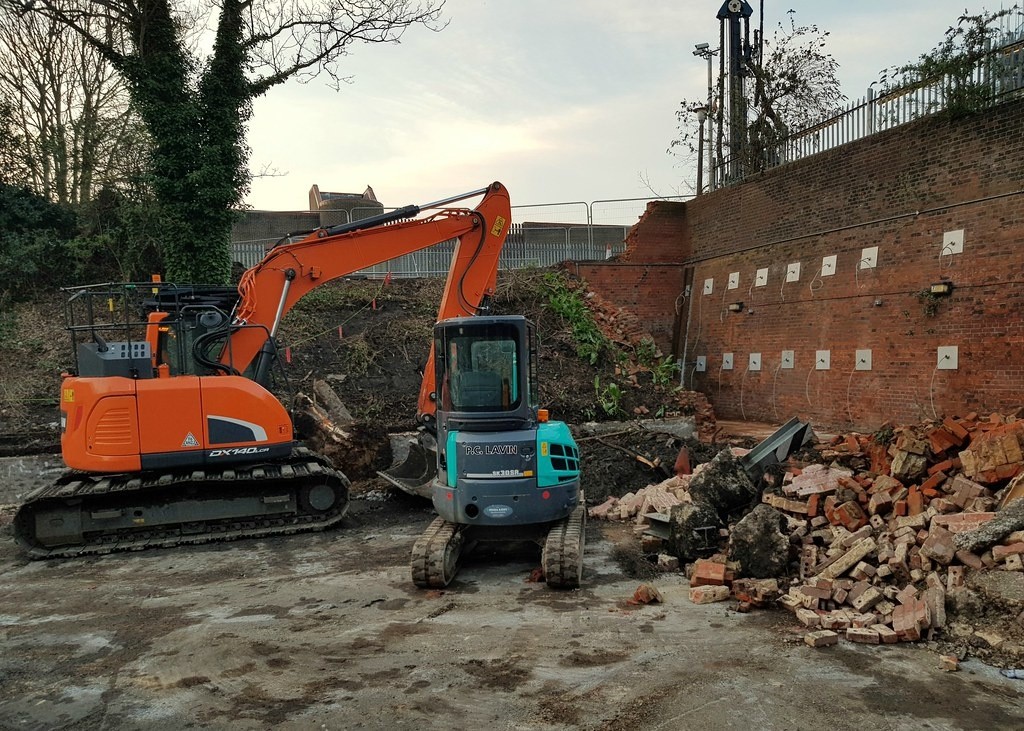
[
  {"x1": 11, "y1": 182, "x2": 512, "y2": 561},
  {"x1": 409, "y1": 314, "x2": 588, "y2": 590}
]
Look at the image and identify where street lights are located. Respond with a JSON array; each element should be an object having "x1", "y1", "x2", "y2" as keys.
[{"x1": 693, "y1": 106, "x2": 709, "y2": 196}]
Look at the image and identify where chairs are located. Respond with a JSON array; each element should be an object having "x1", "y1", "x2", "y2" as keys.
[{"x1": 459, "y1": 372, "x2": 503, "y2": 407}]
